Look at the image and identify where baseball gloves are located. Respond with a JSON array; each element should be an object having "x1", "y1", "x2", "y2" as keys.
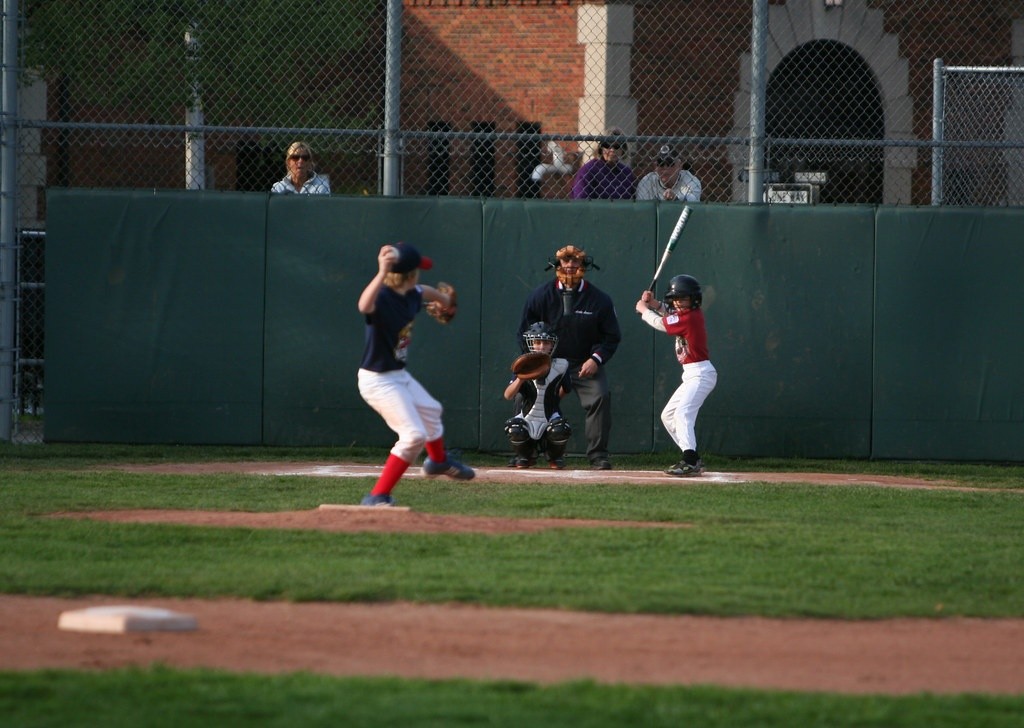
[
  {"x1": 511, "y1": 351, "x2": 551, "y2": 380},
  {"x1": 424, "y1": 281, "x2": 458, "y2": 324}
]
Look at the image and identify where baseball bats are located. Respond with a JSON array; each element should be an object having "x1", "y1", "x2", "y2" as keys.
[{"x1": 636, "y1": 204, "x2": 694, "y2": 316}]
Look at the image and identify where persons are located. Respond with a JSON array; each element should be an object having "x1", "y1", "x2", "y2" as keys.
[
  {"x1": 635, "y1": 145, "x2": 700, "y2": 202},
  {"x1": 504, "y1": 322, "x2": 573, "y2": 469},
  {"x1": 517, "y1": 245, "x2": 621, "y2": 469},
  {"x1": 637, "y1": 273, "x2": 717, "y2": 475},
  {"x1": 358, "y1": 242, "x2": 476, "y2": 505},
  {"x1": 271, "y1": 142, "x2": 330, "y2": 193},
  {"x1": 571, "y1": 127, "x2": 638, "y2": 199}
]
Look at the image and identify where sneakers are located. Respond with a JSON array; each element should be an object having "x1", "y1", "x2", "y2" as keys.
[
  {"x1": 423, "y1": 451, "x2": 477, "y2": 482},
  {"x1": 360, "y1": 493, "x2": 394, "y2": 507},
  {"x1": 699, "y1": 461, "x2": 705, "y2": 472},
  {"x1": 664, "y1": 459, "x2": 701, "y2": 476}
]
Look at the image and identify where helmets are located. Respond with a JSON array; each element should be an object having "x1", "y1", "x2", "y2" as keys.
[
  {"x1": 666, "y1": 274, "x2": 702, "y2": 302},
  {"x1": 524, "y1": 320, "x2": 558, "y2": 341}
]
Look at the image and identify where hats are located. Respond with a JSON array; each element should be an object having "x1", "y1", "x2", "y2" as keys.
[
  {"x1": 656, "y1": 142, "x2": 684, "y2": 160},
  {"x1": 387, "y1": 241, "x2": 431, "y2": 274}
]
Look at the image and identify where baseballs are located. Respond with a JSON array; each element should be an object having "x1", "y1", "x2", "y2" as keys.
[{"x1": 385, "y1": 247, "x2": 400, "y2": 260}]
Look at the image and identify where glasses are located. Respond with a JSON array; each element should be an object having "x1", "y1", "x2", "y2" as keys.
[
  {"x1": 657, "y1": 157, "x2": 676, "y2": 167},
  {"x1": 601, "y1": 142, "x2": 621, "y2": 150},
  {"x1": 290, "y1": 153, "x2": 309, "y2": 160}
]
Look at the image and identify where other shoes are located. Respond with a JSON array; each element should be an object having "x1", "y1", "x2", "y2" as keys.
[
  {"x1": 507, "y1": 455, "x2": 537, "y2": 467},
  {"x1": 590, "y1": 456, "x2": 612, "y2": 470},
  {"x1": 516, "y1": 456, "x2": 530, "y2": 468},
  {"x1": 551, "y1": 459, "x2": 565, "y2": 470}
]
